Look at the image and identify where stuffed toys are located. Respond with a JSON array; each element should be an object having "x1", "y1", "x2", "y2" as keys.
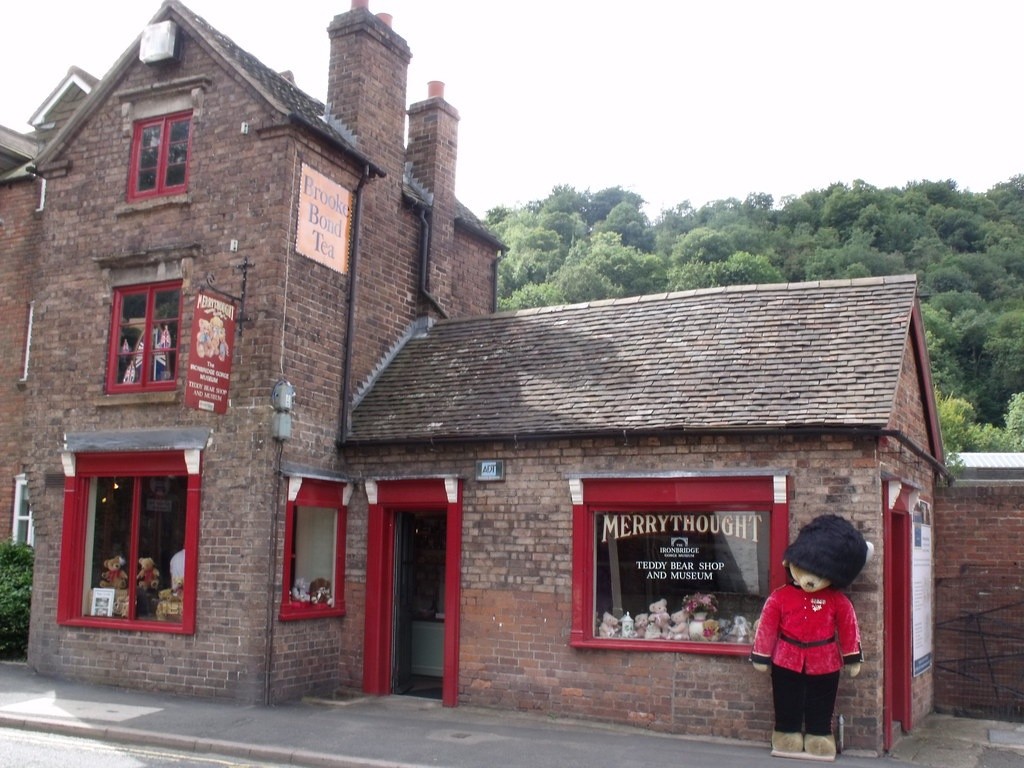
[
  {"x1": 136, "y1": 556, "x2": 160, "y2": 590},
  {"x1": 99, "y1": 556, "x2": 127, "y2": 589},
  {"x1": 750, "y1": 513, "x2": 868, "y2": 754},
  {"x1": 635, "y1": 598, "x2": 761, "y2": 643},
  {"x1": 599, "y1": 611, "x2": 618, "y2": 638}
]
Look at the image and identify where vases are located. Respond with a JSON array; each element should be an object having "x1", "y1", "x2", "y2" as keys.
[{"x1": 690, "y1": 612, "x2": 708, "y2": 641}]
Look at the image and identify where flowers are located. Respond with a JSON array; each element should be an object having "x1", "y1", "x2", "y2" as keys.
[{"x1": 683, "y1": 593, "x2": 719, "y2": 613}]
